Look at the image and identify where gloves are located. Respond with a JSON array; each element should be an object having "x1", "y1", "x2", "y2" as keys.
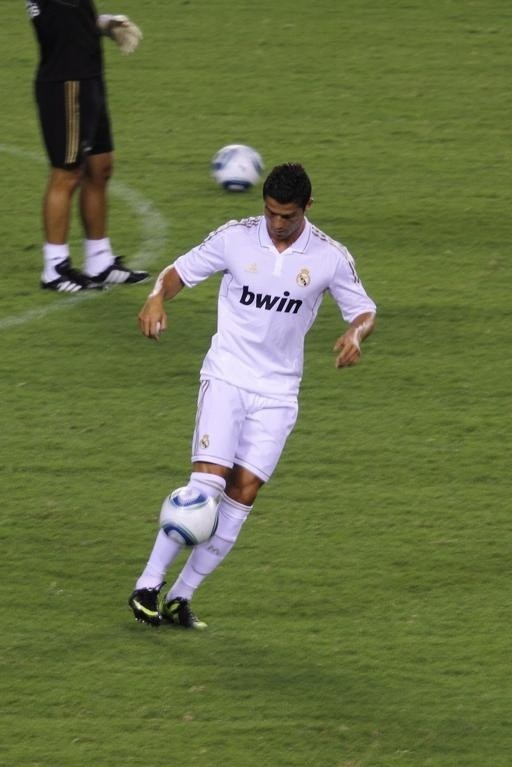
[{"x1": 96, "y1": 14, "x2": 144, "y2": 53}]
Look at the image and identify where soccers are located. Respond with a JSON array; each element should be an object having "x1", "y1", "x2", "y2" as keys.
[
  {"x1": 209, "y1": 144, "x2": 264, "y2": 191},
  {"x1": 160, "y1": 486, "x2": 219, "y2": 545}
]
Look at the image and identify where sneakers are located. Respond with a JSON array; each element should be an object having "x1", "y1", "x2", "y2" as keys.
[
  {"x1": 40, "y1": 256, "x2": 151, "y2": 294},
  {"x1": 128, "y1": 581, "x2": 208, "y2": 631}
]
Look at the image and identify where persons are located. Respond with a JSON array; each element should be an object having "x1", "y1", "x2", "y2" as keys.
[
  {"x1": 25, "y1": 1, "x2": 152, "y2": 295},
  {"x1": 128, "y1": 162, "x2": 377, "y2": 631}
]
